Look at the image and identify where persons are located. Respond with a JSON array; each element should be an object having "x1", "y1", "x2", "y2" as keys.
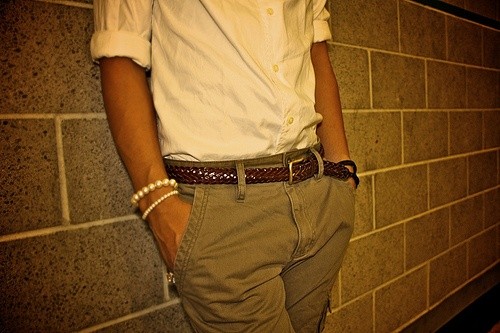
[{"x1": 90, "y1": 1, "x2": 359, "y2": 333}]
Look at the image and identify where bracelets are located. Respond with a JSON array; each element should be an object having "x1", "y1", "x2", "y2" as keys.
[
  {"x1": 130, "y1": 178, "x2": 180, "y2": 221},
  {"x1": 336, "y1": 160, "x2": 360, "y2": 189}
]
